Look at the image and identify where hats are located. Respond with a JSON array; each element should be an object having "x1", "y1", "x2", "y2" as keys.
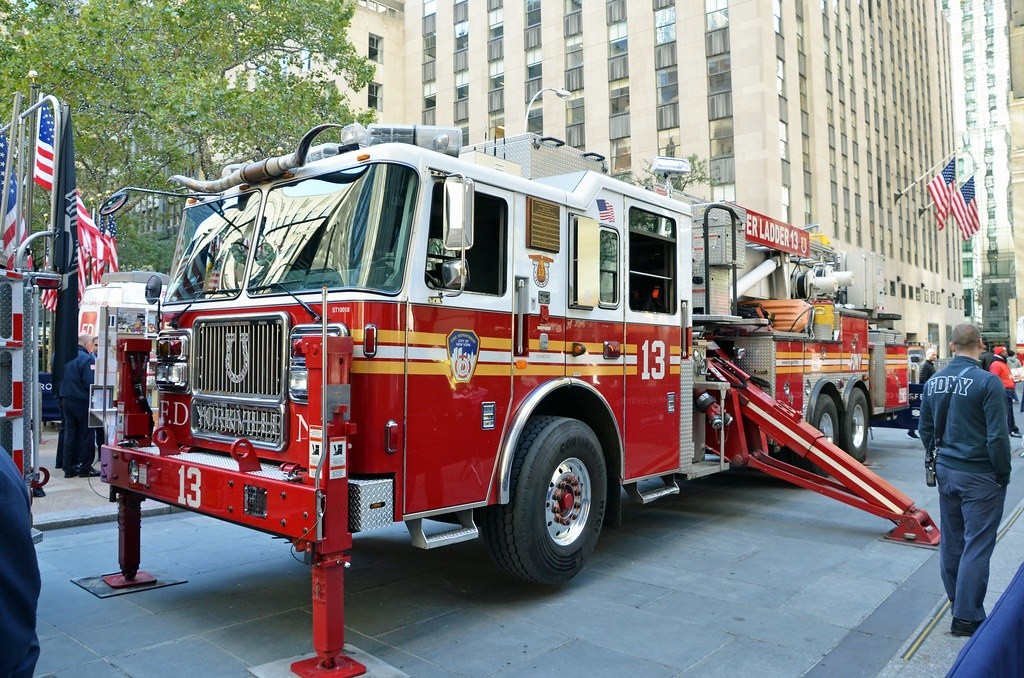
[{"x1": 994, "y1": 346, "x2": 1007, "y2": 355}]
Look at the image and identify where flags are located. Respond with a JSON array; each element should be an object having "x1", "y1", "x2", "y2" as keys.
[
  {"x1": 927, "y1": 158, "x2": 980, "y2": 241},
  {"x1": 0, "y1": 93, "x2": 117, "y2": 312}
]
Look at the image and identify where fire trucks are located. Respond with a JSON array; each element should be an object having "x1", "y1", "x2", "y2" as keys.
[{"x1": 66, "y1": 115, "x2": 944, "y2": 678}]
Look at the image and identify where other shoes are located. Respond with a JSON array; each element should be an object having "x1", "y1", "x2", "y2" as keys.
[
  {"x1": 907, "y1": 432, "x2": 919, "y2": 438},
  {"x1": 1010, "y1": 431, "x2": 1022, "y2": 438},
  {"x1": 951, "y1": 618, "x2": 985, "y2": 636},
  {"x1": 64, "y1": 468, "x2": 80, "y2": 478},
  {"x1": 79, "y1": 466, "x2": 101, "y2": 477}
]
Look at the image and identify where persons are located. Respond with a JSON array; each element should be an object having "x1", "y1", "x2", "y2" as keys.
[
  {"x1": 0, "y1": 445, "x2": 42, "y2": 678},
  {"x1": 51, "y1": 334, "x2": 104, "y2": 479},
  {"x1": 906, "y1": 345, "x2": 1022, "y2": 439},
  {"x1": 918, "y1": 324, "x2": 1012, "y2": 637}
]
